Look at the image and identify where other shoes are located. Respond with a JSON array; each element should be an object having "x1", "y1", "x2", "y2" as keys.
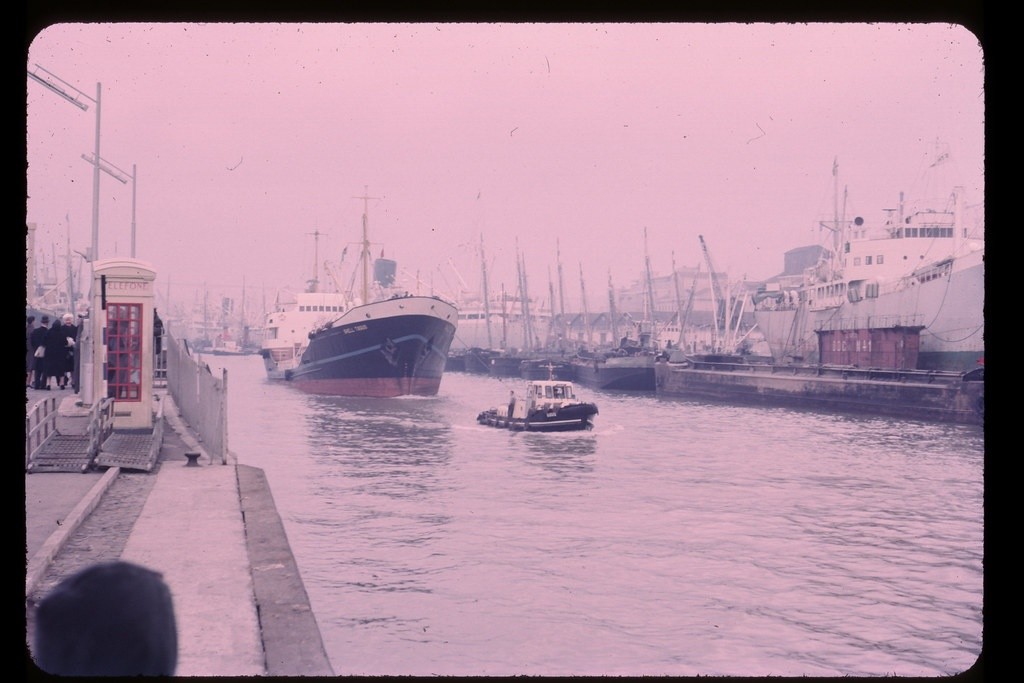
[{"x1": 26, "y1": 377, "x2": 75, "y2": 390}]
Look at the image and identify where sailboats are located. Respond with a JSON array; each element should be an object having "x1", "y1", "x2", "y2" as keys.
[{"x1": 265, "y1": 181, "x2": 460, "y2": 403}]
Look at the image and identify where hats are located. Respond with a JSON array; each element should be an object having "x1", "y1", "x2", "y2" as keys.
[{"x1": 40, "y1": 316, "x2": 50, "y2": 323}]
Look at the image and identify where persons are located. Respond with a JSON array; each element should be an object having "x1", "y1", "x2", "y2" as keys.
[
  {"x1": 32, "y1": 559, "x2": 179, "y2": 676},
  {"x1": 391, "y1": 291, "x2": 413, "y2": 299},
  {"x1": 26, "y1": 308, "x2": 165, "y2": 399},
  {"x1": 554, "y1": 388, "x2": 559, "y2": 399},
  {"x1": 508, "y1": 391, "x2": 517, "y2": 422}
]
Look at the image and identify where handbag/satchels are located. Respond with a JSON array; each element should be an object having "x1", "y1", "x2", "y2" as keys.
[{"x1": 33, "y1": 346, "x2": 45, "y2": 358}]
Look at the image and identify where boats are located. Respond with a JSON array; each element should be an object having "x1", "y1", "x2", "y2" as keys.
[
  {"x1": 478, "y1": 380, "x2": 599, "y2": 431},
  {"x1": 751, "y1": 155, "x2": 986, "y2": 369},
  {"x1": 259, "y1": 220, "x2": 350, "y2": 380},
  {"x1": 170, "y1": 273, "x2": 256, "y2": 357},
  {"x1": 448, "y1": 230, "x2": 756, "y2": 385}
]
